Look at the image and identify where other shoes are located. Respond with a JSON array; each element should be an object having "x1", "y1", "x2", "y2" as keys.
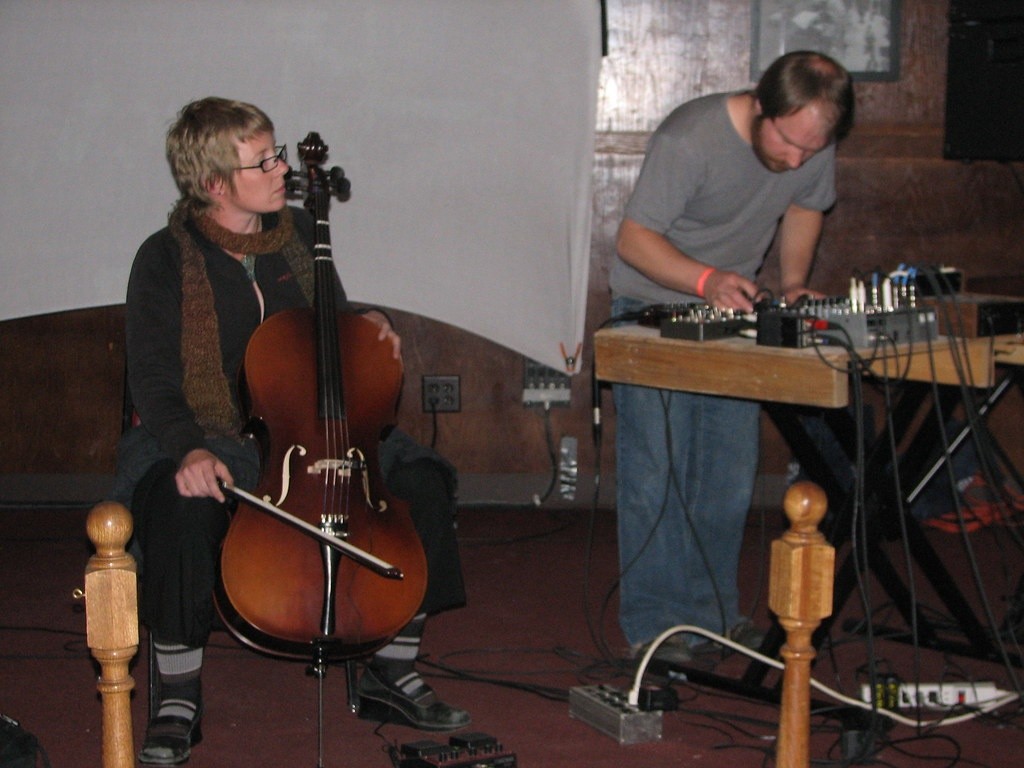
[
  {"x1": 636, "y1": 638, "x2": 692, "y2": 664},
  {"x1": 708, "y1": 628, "x2": 763, "y2": 650}
]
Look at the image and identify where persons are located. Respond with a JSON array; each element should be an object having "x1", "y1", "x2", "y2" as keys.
[
  {"x1": 110, "y1": 98, "x2": 471, "y2": 764},
  {"x1": 606, "y1": 50, "x2": 856, "y2": 667}
]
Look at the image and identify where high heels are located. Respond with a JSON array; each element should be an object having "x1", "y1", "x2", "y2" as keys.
[
  {"x1": 354, "y1": 666, "x2": 472, "y2": 730},
  {"x1": 137, "y1": 692, "x2": 204, "y2": 765}
]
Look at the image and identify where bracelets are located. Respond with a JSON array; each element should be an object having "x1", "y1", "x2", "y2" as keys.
[{"x1": 697, "y1": 266, "x2": 716, "y2": 298}]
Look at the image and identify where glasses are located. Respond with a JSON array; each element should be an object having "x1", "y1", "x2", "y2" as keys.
[{"x1": 211, "y1": 144, "x2": 287, "y2": 173}]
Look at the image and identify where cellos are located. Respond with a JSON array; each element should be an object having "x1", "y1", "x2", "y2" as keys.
[{"x1": 214, "y1": 132, "x2": 429, "y2": 768}]
